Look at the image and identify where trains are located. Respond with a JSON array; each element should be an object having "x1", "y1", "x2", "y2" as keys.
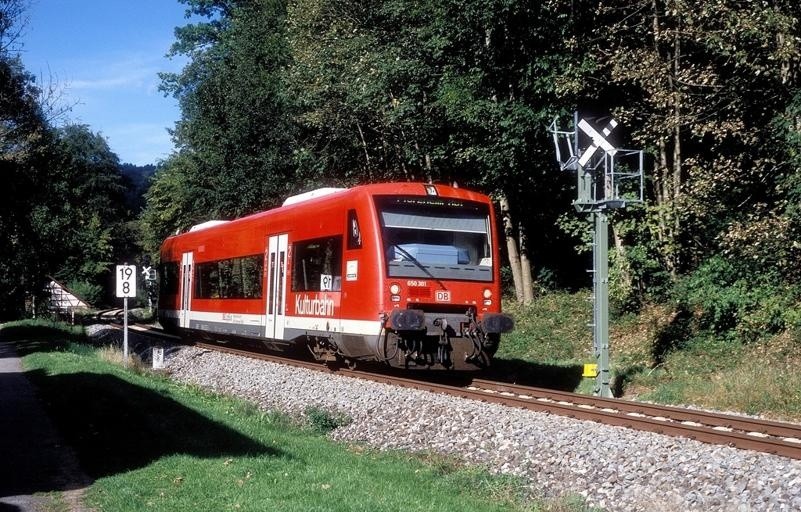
[{"x1": 158, "y1": 181, "x2": 514, "y2": 372}]
